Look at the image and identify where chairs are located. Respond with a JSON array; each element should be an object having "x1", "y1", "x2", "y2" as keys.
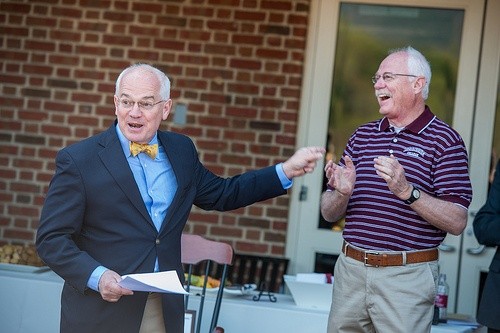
[
  {"x1": 234, "y1": 254, "x2": 290, "y2": 294},
  {"x1": 181, "y1": 233, "x2": 233, "y2": 333}
]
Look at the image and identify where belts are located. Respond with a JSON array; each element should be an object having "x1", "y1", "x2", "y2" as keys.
[{"x1": 341, "y1": 242, "x2": 439, "y2": 268}]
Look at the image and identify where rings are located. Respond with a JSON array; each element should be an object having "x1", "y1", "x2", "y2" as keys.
[{"x1": 391, "y1": 172, "x2": 395, "y2": 177}]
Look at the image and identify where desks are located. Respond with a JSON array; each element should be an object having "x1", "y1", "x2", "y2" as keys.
[{"x1": 0, "y1": 267, "x2": 479, "y2": 333}]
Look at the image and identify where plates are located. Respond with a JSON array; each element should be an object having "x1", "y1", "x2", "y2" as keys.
[
  {"x1": 0, "y1": 263, "x2": 50, "y2": 273},
  {"x1": 184, "y1": 285, "x2": 219, "y2": 292}
]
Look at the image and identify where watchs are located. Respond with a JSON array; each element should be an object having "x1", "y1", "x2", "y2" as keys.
[{"x1": 402, "y1": 185, "x2": 420, "y2": 205}]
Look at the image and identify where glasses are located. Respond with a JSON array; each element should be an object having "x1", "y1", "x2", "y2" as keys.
[
  {"x1": 120, "y1": 98, "x2": 167, "y2": 110},
  {"x1": 371, "y1": 73, "x2": 417, "y2": 84}
]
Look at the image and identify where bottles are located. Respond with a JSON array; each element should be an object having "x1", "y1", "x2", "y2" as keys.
[{"x1": 436, "y1": 274, "x2": 449, "y2": 323}]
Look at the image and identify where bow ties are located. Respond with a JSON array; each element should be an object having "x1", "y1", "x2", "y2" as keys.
[{"x1": 130, "y1": 142, "x2": 158, "y2": 160}]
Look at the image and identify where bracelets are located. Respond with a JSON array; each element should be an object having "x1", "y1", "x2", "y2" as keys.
[{"x1": 335, "y1": 189, "x2": 345, "y2": 197}]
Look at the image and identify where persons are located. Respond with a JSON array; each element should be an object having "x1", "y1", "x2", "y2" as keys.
[
  {"x1": 472, "y1": 159, "x2": 500, "y2": 333},
  {"x1": 320, "y1": 46, "x2": 472, "y2": 333},
  {"x1": 36, "y1": 64, "x2": 326, "y2": 333}
]
